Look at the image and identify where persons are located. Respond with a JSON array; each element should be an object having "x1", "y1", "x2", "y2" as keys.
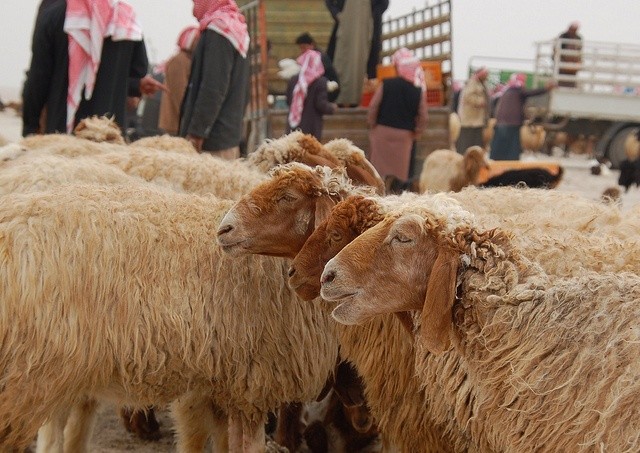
[
  {"x1": 22, "y1": 0, "x2": 148, "y2": 140},
  {"x1": 552, "y1": 22, "x2": 583, "y2": 87},
  {"x1": 456, "y1": 58, "x2": 493, "y2": 153},
  {"x1": 157, "y1": 26, "x2": 200, "y2": 134},
  {"x1": 126, "y1": 77, "x2": 172, "y2": 99},
  {"x1": 366, "y1": 57, "x2": 426, "y2": 182},
  {"x1": 178, "y1": 1, "x2": 251, "y2": 158},
  {"x1": 286, "y1": 50, "x2": 336, "y2": 141},
  {"x1": 490, "y1": 72, "x2": 556, "y2": 160},
  {"x1": 285, "y1": 33, "x2": 344, "y2": 104},
  {"x1": 392, "y1": 47, "x2": 417, "y2": 177}
]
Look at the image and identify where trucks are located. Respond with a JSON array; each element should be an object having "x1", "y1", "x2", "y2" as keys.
[
  {"x1": 468, "y1": 37, "x2": 640, "y2": 169},
  {"x1": 235, "y1": 0, "x2": 453, "y2": 180}
]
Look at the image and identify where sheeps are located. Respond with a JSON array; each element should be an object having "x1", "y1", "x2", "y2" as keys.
[
  {"x1": 288, "y1": 194, "x2": 639, "y2": 343},
  {"x1": 319, "y1": 204, "x2": 640, "y2": 451},
  {"x1": 0, "y1": 112, "x2": 387, "y2": 453},
  {"x1": 216, "y1": 160, "x2": 640, "y2": 452},
  {"x1": 418, "y1": 144, "x2": 490, "y2": 194}
]
